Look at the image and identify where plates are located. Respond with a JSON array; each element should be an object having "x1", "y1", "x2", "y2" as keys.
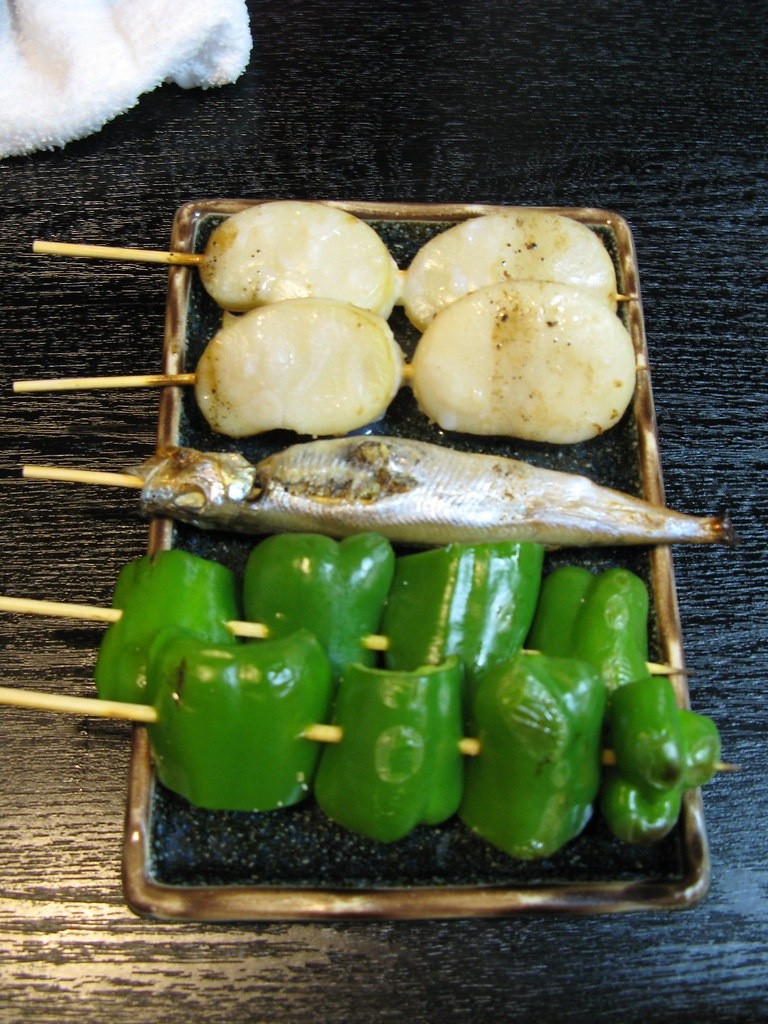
[{"x1": 119, "y1": 197, "x2": 710, "y2": 923}]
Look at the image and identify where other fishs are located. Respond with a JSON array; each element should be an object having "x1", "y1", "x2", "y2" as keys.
[{"x1": 120, "y1": 437, "x2": 736, "y2": 551}]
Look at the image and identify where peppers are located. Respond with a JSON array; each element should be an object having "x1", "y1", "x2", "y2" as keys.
[{"x1": 94, "y1": 531, "x2": 721, "y2": 858}]
